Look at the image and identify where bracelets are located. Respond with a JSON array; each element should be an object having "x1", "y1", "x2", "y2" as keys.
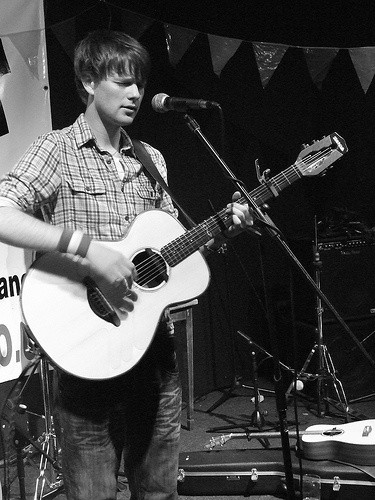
[{"x1": 57, "y1": 230, "x2": 93, "y2": 258}]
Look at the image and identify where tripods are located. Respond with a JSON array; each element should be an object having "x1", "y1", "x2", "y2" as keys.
[
  {"x1": 0, "y1": 361, "x2": 64, "y2": 500},
  {"x1": 161, "y1": 117, "x2": 353, "y2": 452}
]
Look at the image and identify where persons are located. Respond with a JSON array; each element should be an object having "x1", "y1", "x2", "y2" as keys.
[{"x1": 0, "y1": 29, "x2": 252, "y2": 500}]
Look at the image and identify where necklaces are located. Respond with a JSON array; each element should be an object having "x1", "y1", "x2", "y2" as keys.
[{"x1": 112, "y1": 144, "x2": 120, "y2": 155}]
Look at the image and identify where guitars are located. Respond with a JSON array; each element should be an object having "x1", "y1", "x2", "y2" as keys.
[
  {"x1": 19, "y1": 129, "x2": 353, "y2": 385},
  {"x1": 203, "y1": 418, "x2": 374, "y2": 467}
]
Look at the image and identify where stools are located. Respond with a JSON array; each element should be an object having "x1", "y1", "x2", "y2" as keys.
[{"x1": 168, "y1": 299, "x2": 198, "y2": 431}]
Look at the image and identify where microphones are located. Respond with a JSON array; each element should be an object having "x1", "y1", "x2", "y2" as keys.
[{"x1": 151, "y1": 93, "x2": 219, "y2": 112}]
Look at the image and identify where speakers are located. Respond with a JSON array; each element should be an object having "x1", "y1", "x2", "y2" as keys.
[
  {"x1": 289, "y1": 240, "x2": 375, "y2": 325},
  {"x1": 278, "y1": 322, "x2": 375, "y2": 404},
  {"x1": 178, "y1": 244, "x2": 277, "y2": 400}
]
